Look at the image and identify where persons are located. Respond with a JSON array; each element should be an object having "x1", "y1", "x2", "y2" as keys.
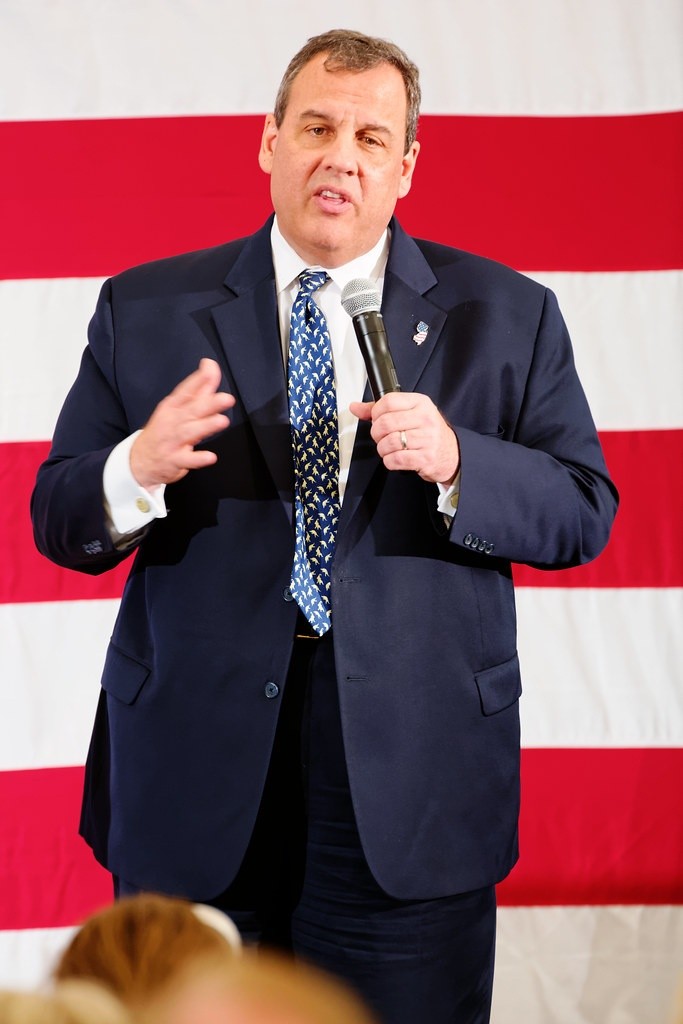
[
  {"x1": 28, "y1": 29, "x2": 622, "y2": 1024},
  {"x1": 0, "y1": 891, "x2": 370, "y2": 1024}
]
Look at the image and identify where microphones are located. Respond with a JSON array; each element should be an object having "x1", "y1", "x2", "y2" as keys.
[{"x1": 339, "y1": 279, "x2": 403, "y2": 404}]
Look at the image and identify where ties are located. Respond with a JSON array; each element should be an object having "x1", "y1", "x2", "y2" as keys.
[{"x1": 287, "y1": 270, "x2": 339, "y2": 637}]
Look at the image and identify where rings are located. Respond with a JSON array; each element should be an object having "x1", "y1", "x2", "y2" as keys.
[{"x1": 400, "y1": 430, "x2": 407, "y2": 449}]
